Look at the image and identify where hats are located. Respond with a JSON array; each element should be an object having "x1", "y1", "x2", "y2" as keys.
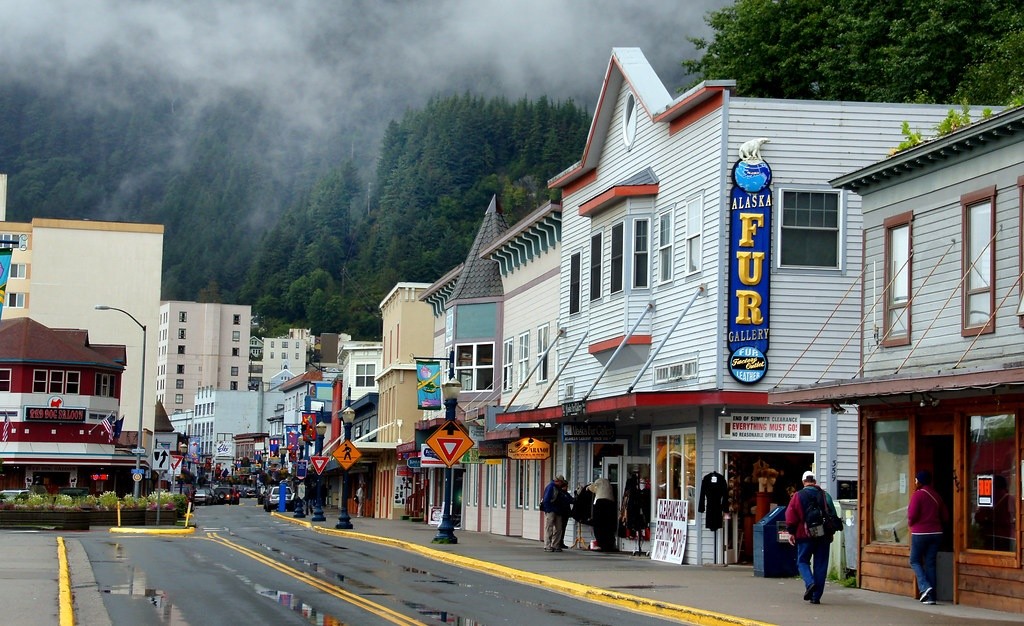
[
  {"x1": 554, "y1": 474, "x2": 566, "y2": 484},
  {"x1": 915, "y1": 472, "x2": 931, "y2": 486},
  {"x1": 802, "y1": 471, "x2": 815, "y2": 481}
]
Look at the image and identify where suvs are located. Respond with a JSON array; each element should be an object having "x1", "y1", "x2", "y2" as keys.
[{"x1": 213, "y1": 486, "x2": 240, "y2": 505}]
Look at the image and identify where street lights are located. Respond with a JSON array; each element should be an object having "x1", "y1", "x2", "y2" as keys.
[
  {"x1": 280, "y1": 446, "x2": 287, "y2": 469},
  {"x1": 433, "y1": 376, "x2": 463, "y2": 544},
  {"x1": 94, "y1": 305, "x2": 146, "y2": 497},
  {"x1": 293, "y1": 435, "x2": 307, "y2": 518},
  {"x1": 312, "y1": 421, "x2": 327, "y2": 522},
  {"x1": 335, "y1": 405, "x2": 355, "y2": 529}
]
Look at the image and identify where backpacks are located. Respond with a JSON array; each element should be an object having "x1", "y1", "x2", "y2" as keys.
[{"x1": 799, "y1": 489, "x2": 834, "y2": 544}]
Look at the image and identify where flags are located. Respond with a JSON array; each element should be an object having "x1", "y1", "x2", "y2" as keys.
[
  {"x1": 2, "y1": 413, "x2": 10, "y2": 442},
  {"x1": 101, "y1": 414, "x2": 113, "y2": 434},
  {"x1": 112, "y1": 417, "x2": 125, "y2": 439}
]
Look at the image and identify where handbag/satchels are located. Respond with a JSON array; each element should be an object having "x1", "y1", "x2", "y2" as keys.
[
  {"x1": 353, "y1": 495, "x2": 359, "y2": 504},
  {"x1": 540, "y1": 499, "x2": 545, "y2": 511}
]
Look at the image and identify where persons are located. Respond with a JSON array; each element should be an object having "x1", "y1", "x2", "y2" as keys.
[
  {"x1": 785, "y1": 471, "x2": 838, "y2": 604},
  {"x1": 905, "y1": 470, "x2": 948, "y2": 605},
  {"x1": 356, "y1": 482, "x2": 363, "y2": 518},
  {"x1": 973, "y1": 476, "x2": 1018, "y2": 552},
  {"x1": 628, "y1": 478, "x2": 651, "y2": 530},
  {"x1": 540, "y1": 475, "x2": 574, "y2": 552},
  {"x1": 756, "y1": 468, "x2": 779, "y2": 492}
]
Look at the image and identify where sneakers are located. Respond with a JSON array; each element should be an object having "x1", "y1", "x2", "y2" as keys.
[
  {"x1": 920, "y1": 587, "x2": 933, "y2": 602},
  {"x1": 922, "y1": 598, "x2": 936, "y2": 605},
  {"x1": 804, "y1": 584, "x2": 817, "y2": 601},
  {"x1": 810, "y1": 599, "x2": 819, "y2": 603}
]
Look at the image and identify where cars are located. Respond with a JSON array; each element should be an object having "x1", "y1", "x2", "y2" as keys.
[
  {"x1": 60, "y1": 487, "x2": 90, "y2": 504},
  {"x1": 193, "y1": 488, "x2": 214, "y2": 506},
  {"x1": 0, "y1": 489, "x2": 29, "y2": 502},
  {"x1": 258, "y1": 484, "x2": 296, "y2": 513},
  {"x1": 232, "y1": 485, "x2": 257, "y2": 498}
]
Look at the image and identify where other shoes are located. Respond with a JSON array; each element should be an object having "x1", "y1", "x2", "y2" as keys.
[
  {"x1": 357, "y1": 515, "x2": 364, "y2": 517},
  {"x1": 544, "y1": 547, "x2": 553, "y2": 552},
  {"x1": 561, "y1": 544, "x2": 568, "y2": 549},
  {"x1": 553, "y1": 548, "x2": 561, "y2": 552}
]
